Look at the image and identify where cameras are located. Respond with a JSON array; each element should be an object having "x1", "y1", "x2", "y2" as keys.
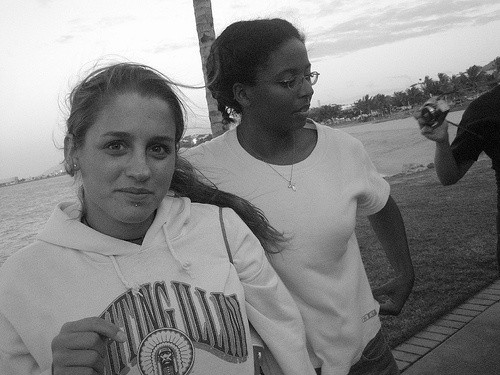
[{"x1": 420, "y1": 97, "x2": 450, "y2": 129}]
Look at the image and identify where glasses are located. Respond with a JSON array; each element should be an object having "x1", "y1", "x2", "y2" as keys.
[{"x1": 243, "y1": 71, "x2": 319, "y2": 90}]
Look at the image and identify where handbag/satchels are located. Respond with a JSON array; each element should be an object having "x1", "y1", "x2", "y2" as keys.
[{"x1": 250, "y1": 329, "x2": 264, "y2": 374}]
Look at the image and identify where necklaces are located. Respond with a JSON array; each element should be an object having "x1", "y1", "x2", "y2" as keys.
[{"x1": 239, "y1": 122, "x2": 299, "y2": 190}]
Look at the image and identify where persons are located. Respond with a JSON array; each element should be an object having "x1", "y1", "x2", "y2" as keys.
[
  {"x1": 178, "y1": 17, "x2": 415, "y2": 374},
  {"x1": 0, "y1": 63, "x2": 315, "y2": 375},
  {"x1": 415, "y1": 82, "x2": 500, "y2": 236}
]
